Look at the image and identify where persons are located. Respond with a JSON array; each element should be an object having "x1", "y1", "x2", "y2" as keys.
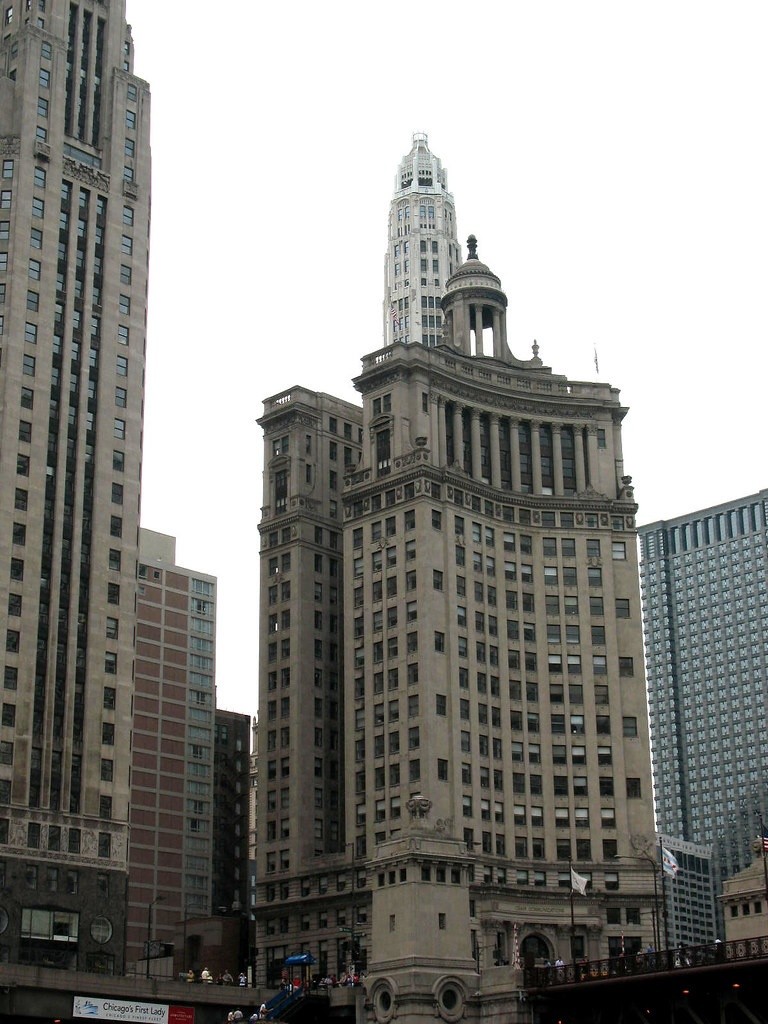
[
  {"x1": 554, "y1": 957, "x2": 564, "y2": 969},
  {"x1": 187, "y1": 970, "x2": 195, "y2": 982},
  {"x1": 635, "y1": 948, "x2": 646, "y2": 967},
  {"x1": 544, "y1": 960, "x2": 551, "y2": 968},
  {"x1": 238, "y1": 973, "x2": 247, "y2": 987},
  {"x1": 228, "y1": 1001, "x2": 274, "y2": 1024},
  {"x1": 582, "y1": 955, "x2": 589, "y2": 971},
  {"x1": 279, "y1": 972, "x2": 365, "y2": 990},
  {"x1": 678, "y1": 941, "x2": 689, "y2": 964},
  {"x1": 617, "y1": 950, "x2": 626, "y2": 969},
  {"x1": 202, "y1": 967, "x2": 233, "y2": 986},
  {"x1": 646, "y1": 943, "x2": 654, "y2": 961},
  {"x1": 714, "y1": 937, "x2": 721, "y2": 950}
]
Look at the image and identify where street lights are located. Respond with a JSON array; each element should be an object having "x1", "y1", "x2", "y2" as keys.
[
  {"x1": 184, "y1": 903, "x2": 228, "y2": 972},
  {"x1": 146, "y1": 894, "x2": 167, "y2": 978},
  {"x1": 613, "y1": 855, "x2": 662, "y2": 952}
]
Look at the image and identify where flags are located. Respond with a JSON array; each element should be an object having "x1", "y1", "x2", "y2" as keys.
[
  {"x1": 763, "y1": 824, "x2": 768, "y2": 851},
  {"x1": 512, "y1": 927, "x2": 520, "y2": 965},
  {"x1": 571, "y1": 867, "x2": 588, "y2": 897},
  {"x1": 662, "y1": 846, "x2": 679, "y2": 878}
]
